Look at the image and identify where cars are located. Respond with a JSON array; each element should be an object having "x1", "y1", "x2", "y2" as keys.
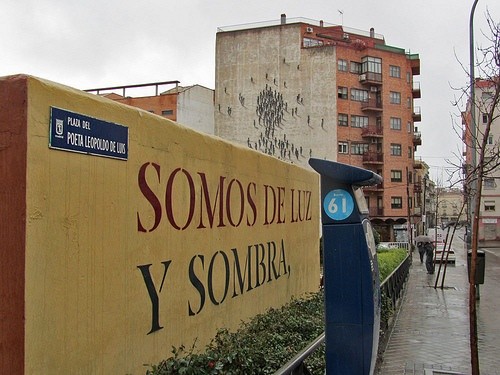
[
  {"x1": 433, "y1": 245, "x2": 455, "y2": 265},
  {"x1": 433, "y1": 233, "x2": 445, "y2": 247}
]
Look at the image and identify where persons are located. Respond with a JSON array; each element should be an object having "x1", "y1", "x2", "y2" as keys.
[
  {"x1": 425, "y1": 242, "x2": 435, "y2": 258},
  {"x1": 417, "y1": 242, "x2": 426, "y2": 262}
]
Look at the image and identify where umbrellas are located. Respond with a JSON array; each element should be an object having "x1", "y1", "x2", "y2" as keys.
[{"x1": 414, "y1": 235, "x2": 434, "y2": 242}]
